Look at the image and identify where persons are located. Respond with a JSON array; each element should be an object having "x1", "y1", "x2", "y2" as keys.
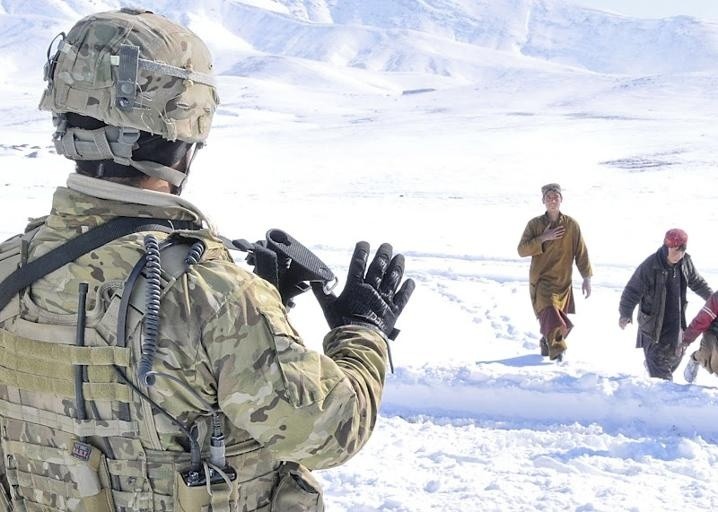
[
  {"x1": 518, "y1": 183, "x2": 593, "y2": 365},
  {"x1": 676, "y1": 290, "x2": 718, "y2": 382},
  {"x1": 620, "y1": 228, "x2": 713, "y2": 383},
  {"x1": 1, "y1": 7, "x2": 414, "y2": 512}
]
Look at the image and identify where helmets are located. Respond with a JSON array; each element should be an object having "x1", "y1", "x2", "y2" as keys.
[{"x1": 38, "y1": 6, "x2": 221, "y2": 145}]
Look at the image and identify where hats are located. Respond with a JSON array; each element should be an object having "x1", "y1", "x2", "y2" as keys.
[
  {"x1": 541, "y1": 183, "x2": 561, "y2": 197},
  {"x1": 665, "y1": 229, "x2": 688, "y2": 247}
]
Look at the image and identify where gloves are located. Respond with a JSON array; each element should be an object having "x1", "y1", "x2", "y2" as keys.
[{"x1": 311, "y1": 240, "x2": 416, "y2": 338}]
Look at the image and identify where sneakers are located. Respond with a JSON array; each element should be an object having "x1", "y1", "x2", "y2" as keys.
[{"x1": 684, "y1": 354, "x2": 699, "y2": 382}]
